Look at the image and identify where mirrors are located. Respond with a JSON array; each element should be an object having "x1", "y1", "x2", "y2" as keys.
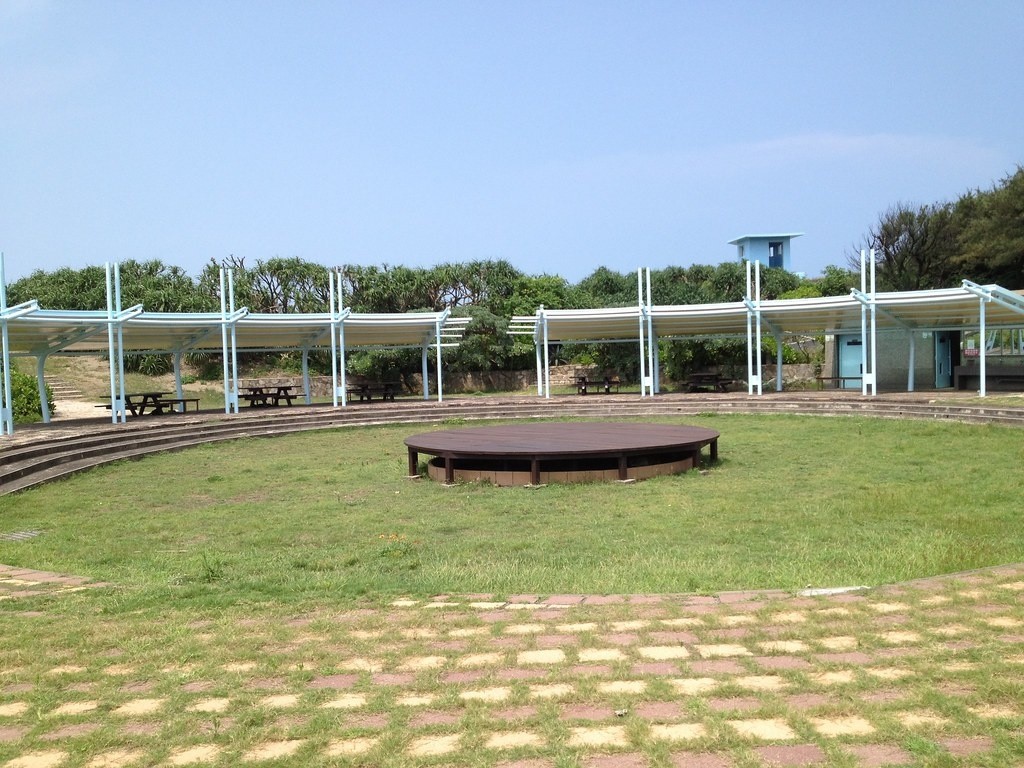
[{"x1": 963, "y1": 327, "x2": 1024, "y2": 357}]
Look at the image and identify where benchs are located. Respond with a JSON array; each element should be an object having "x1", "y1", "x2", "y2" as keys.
[
  {"x1": 681, "y1": 379, "x2": 732, "y2": 386},
  {"x1": 95, "y1": 398, "x2": 200, "y2": 412},
  {"x1": 238, "y1": 393, "x2": 307, "y2": 400},
  {"x1": 346, "y1": 389, "x2": 405, "y2": 395},
  {"x1": 571, "y1": 381, "x2": 619, "y2": 387}
]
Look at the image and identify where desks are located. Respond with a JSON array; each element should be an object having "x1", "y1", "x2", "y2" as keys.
[
  {"x1": 816, "y1": 377, "x2": 862, "y2": 391},
  {"x1": 348, "y1": 381, "x2": 401, "y2": 403},
  {"x1": 569, "y1": 376, "x2": 619, "y2": 396},
  {"x1": 238, "y1": 386, "x2": 301, "y2": 408},
  {"x1": 687, "y1": 373, "x2": 727, "y2": 393},
  {"x1": 100, "y1": 392, "x2": 172, "y2": 417}
]
